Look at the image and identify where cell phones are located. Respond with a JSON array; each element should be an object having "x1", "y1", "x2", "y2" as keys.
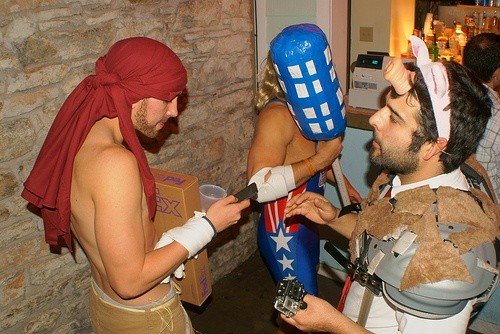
[{"x1": 233, "y1": 182, "x2": 258, "y2": 203}]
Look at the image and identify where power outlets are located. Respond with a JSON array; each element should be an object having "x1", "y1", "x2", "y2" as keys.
[{"x1": 360, "y1": 26, "x2": 373, "y2": 42}]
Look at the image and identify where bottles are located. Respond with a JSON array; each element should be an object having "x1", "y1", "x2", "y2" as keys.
[
  {"x1": 407, "y1": 0, "x2": 500, "y2": 62},
  {"x1": 198, "y1": 185, "x2": 227, "y2": 213}
]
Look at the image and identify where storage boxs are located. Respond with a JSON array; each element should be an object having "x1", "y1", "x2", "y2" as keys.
[{"x1": 149, "y1": 168, "x2": 213, "y2": 307}]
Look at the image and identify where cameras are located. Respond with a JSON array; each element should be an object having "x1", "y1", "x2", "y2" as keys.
[{"x1": 273, "y1": 279, "x2": 308, "y2": 318}]
